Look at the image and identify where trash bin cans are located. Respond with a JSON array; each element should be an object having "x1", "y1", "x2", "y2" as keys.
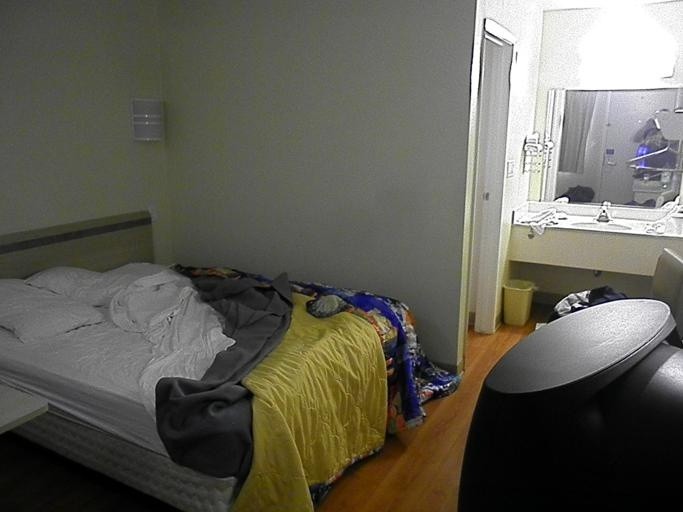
[{"x1": 501, "y1": 280, "x2": 534, "y2": 326}]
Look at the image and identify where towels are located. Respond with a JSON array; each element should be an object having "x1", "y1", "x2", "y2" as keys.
[
  {"x1": 545, "y1": 142, "x2": 553, "y2": 149},
  {"x1": 526, "y1": 133, "x2": 539, "y2": 144},
  {"x1": 520, "y1": 208, "x2": 559, "y2": 237},
  {"x1": 553, "y1": 197, "x2": 569, "y2": 203},
  {"x1": 524, "y1": 144, "x2": 542, "y2": 152}
]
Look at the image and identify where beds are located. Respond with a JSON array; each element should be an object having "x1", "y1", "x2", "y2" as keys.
[{"x1": 0, "y1": 209, "x2": 463, "y2": 512}]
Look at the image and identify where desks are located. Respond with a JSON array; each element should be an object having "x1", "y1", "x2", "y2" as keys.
[{"x1": 0, "y1": 384, "x2": 48, "y2": 433}]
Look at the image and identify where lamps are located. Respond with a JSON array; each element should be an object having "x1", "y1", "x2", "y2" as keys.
[{"x1": 130, "y1": 97, "x2": 166, "y2": 142}]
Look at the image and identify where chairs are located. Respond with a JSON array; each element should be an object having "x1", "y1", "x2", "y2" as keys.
[
  {"x1": 655, "y1": 190, "x2": 675, "y2": 209},
  {"x1": 649, "y1": 248, "x2": 682, "y2": 349}
]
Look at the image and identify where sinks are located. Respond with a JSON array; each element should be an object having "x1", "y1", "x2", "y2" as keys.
[{"x1": 571, "y1": 223, "x2": 633, "y2": 231}]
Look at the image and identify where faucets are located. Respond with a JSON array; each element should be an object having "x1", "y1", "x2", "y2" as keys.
[
  {"x1": 596, "y1": 209, "x2": 611, "y2": 222},
  {"x1": 602, "y1": 201, "x2": 612, "y2": 208}
]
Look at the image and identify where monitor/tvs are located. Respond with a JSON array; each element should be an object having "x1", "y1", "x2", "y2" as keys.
[{"x1": 634, "y1": 144, "x2": 675, "y2": 178}]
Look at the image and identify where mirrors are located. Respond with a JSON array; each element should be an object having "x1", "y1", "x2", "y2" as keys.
[{"x1": 555, "y1": 87, "x2": 683, "y2": 208}]
